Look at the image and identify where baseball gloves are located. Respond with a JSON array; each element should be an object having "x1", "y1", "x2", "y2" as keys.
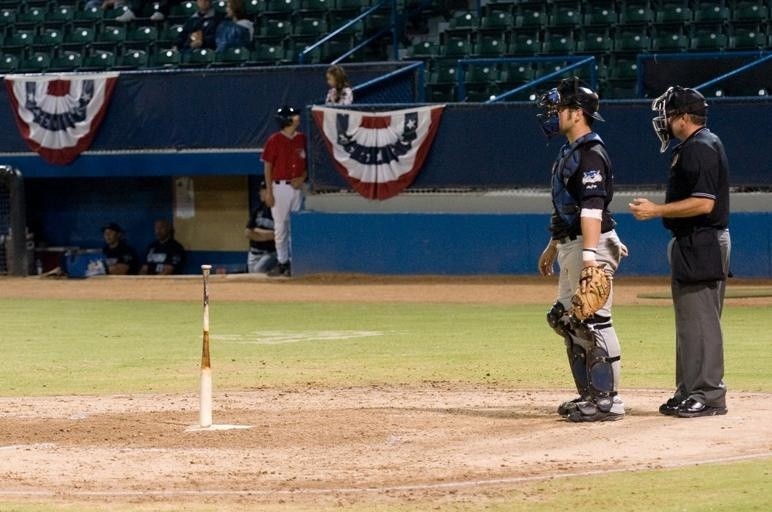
[{"x1": 570, "y1": 264, "x2": 611, "y2": 321}]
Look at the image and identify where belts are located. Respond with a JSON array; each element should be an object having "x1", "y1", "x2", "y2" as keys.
[{"x1": 275, "y1": 180, "x2": 293, "y2": 185}]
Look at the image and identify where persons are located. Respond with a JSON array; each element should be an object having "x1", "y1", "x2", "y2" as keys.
[
  {"x1": 84, "y1": 0, "x2": 256, "y2": 52},
  {"x1": 535, "y1": 76, "x2": 625, "y2": 421},
  {"x1": 260, "y1": 106, "x2": 308, "y2": 275},
  {"x1": 628, "y1": 86, "x2": 733, "y2": 415},
  {"x1": 325, "y1": 65, "x2": 354, "y2": 104},
  {"x1": 138, "y1": 220, "x2": 186, "y2": 274},
  {"x1": 100, "y1": 221, "x2": 137, "y2": 275},
  {"x1": 245, "y1": 180, "x2": 278, "y2": 272}
]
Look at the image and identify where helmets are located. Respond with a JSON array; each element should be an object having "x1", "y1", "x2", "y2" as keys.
[
  {"x1": 650, "y1": 83, "x2": 712, "y2": 153},
  {"x1": 559, "y1": 69, "x2": 615, "y2": 124},
  {"x1": 275, "y1": 105, "x2": 303, "y2": 116}
]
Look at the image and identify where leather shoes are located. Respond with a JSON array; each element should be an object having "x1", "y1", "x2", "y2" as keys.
[
  {"x1": 658, "y1": 395, "x2": 687, "y2": 415},
  {"x1": 672, "y1": 395, "x2": 730, "y2": 418}
]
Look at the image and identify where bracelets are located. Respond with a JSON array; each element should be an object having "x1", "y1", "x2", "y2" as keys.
[{"x1": 583, "y1": 249, "x2": 597, "y2": 261}]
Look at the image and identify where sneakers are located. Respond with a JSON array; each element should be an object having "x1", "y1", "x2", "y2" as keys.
[
  {"x1": 558, "y1": 393, "x2": 586, "y2": 418},
  {"x1": 267, "y1": 262, "x2": 290, "y2": 277}
]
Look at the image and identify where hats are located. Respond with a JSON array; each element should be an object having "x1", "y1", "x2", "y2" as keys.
[{"x1": 100, "y1": 223, "x2": 122, "y2": 233}]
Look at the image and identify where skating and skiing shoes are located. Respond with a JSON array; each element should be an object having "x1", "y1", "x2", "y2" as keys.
[{"x1": 568, "y1": 399, "x2": 626, "y2": 425}]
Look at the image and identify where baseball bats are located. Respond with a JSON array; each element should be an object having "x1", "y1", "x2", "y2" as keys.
[{"x1": 199, "y1": 265, "x2": 213, "y2": 427}]
